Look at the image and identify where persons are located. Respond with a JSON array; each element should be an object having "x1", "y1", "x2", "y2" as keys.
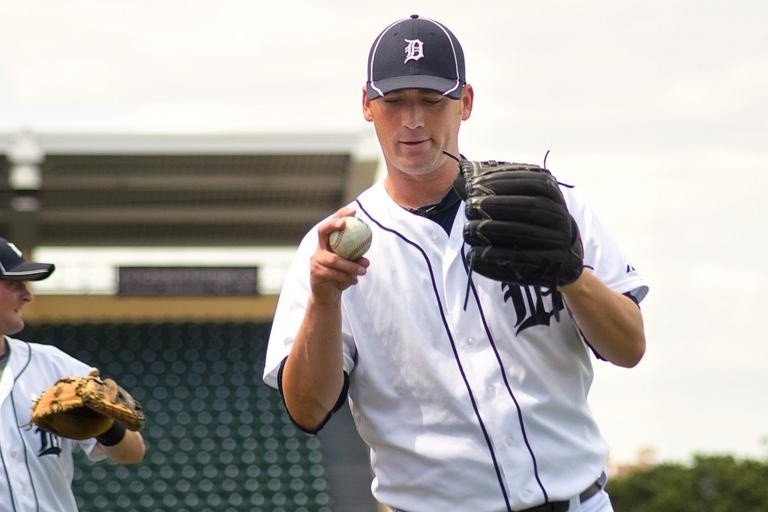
[
  {"x1": 261, "y1": 15, "x2": 646, "y2": 512},
  {"x1": 1, "y1": 235, "x2": 146, "y2": 512}
]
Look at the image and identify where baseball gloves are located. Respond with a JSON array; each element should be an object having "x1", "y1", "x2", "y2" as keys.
[
  {"x1": 30, "y1": 376, "x2": 145, "y2": 440},
  {"x1": 458, "y1": 160, "x2": 584, "y2": 287}
]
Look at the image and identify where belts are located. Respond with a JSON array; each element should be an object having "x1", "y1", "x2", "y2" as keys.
[{"x1": 520, "y1": 470, "x2": 609, "y2": 512}]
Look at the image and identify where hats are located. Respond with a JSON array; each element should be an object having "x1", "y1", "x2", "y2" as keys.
[
  {"x1": 366, "y1": 13, "x2": 467, "y2": 102},
  {"x1": 0, "y1": 237, "x2": 55, "y2": 282}
]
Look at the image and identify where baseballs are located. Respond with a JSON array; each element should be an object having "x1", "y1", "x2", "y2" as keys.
[{"x1": 328, "y1": 216, "x2": 372, "y2": 262}]
